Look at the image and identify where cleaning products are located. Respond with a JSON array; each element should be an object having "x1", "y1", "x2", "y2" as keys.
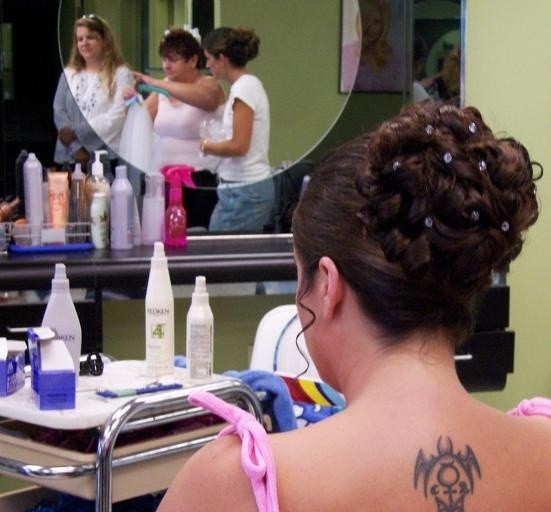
[
  {"x1": 164, "y1": 166, "x2": 197, "y2": 247},
  {"x1": 159, "y1": 163, "x2": 190, "y2": 172},
  {"x1": 85, "y1": 148, "x2": 111, "y2": 215}
]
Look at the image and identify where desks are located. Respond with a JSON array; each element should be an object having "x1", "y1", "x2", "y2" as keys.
[
  {"x1": 0, "y1": 360, "x2": 274, "y2": 512},
  {"x1": 0, "y1": 237, "x2": 298, "y2": 373}
]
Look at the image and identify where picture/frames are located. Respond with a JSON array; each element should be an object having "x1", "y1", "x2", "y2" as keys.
[{"x1": 340, "y1": 0, "x2": 417, "y2": 94}]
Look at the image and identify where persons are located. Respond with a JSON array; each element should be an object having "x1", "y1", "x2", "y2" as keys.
[
  {"x1": 50, "y1": 15, "x2": 145, "y2": 187},
  {"x1": 153, "y1": 93, "x2": 551, "y2": 511},
  {"x1": 197, "y1": 25, "x2": 277, "y2": 234},
  {"x1": 342, "y1": 0, "x2": 408, "y2": 93},
  {"x1": 120, "y1": 24, "x2": 228, "y2": 230},
  {"x1": 409, "y1": 33, "x2": 457, "y2": 113}
]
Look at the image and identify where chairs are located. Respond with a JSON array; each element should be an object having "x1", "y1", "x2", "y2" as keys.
[{"x1": 249, "y1": 304, "x2": 325, "y2": 383}]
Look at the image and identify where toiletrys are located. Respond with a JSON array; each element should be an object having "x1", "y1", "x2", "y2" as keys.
[{"x1": 46, "y1": 171, "x2": 69, "y2": 230}]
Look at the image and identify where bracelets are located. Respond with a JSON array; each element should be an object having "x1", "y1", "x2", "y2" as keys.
[{"x1": 203, "y1": 138, "x2": 208, "y2": 157}]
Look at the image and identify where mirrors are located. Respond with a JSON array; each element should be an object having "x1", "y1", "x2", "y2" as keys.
[{"x1": 56, "y1": 1, "x2": 363, "y2": 190}]
